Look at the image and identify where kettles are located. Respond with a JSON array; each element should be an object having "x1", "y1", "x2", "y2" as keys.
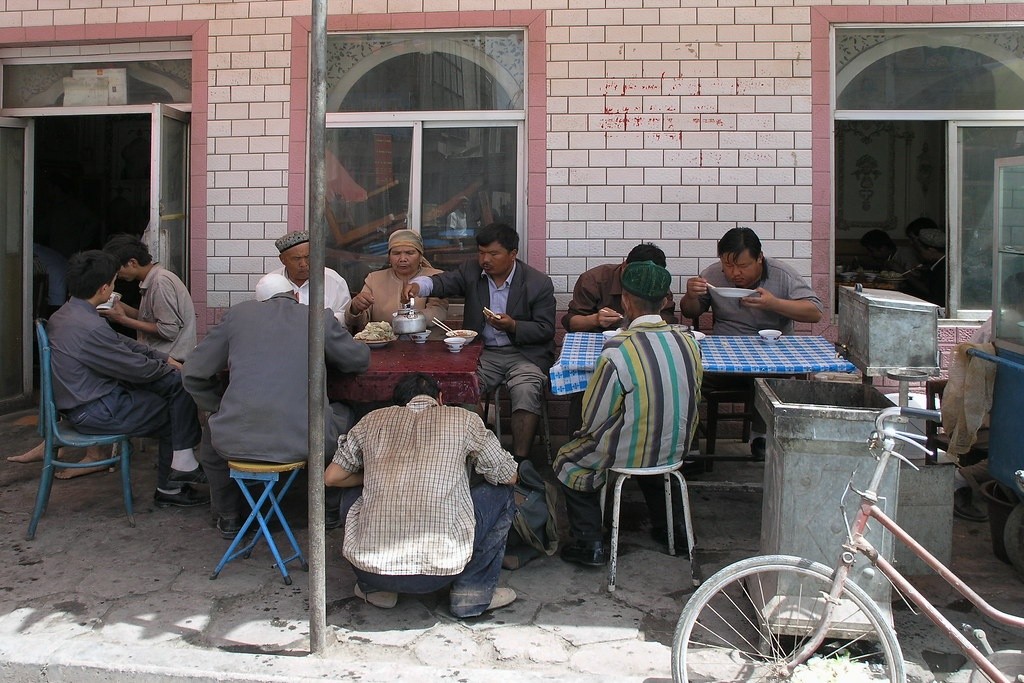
[{"x1": 393, "y1": 295, "x2": 425, "y2": 335}]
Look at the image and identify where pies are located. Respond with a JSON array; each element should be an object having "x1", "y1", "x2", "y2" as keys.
[{"x1": 481, "y1": 308, "x2": 502, "y2": 319}]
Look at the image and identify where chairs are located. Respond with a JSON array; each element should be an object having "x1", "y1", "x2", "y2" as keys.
[
  {"x1": 685, "y1": 304, "x2": 753, "y2": 472},
  {"x1": 922, "y1": 378, "x2": 991, "y2": 498},
  {"x1": 24, "y1": 321, "x2": 137, "y2": 540}
]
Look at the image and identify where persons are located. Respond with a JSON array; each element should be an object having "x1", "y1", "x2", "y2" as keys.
[
  {"x1": 344, "y1": 229, "x2": 450, "y2": 330},
  {"x1": 45, "y1": 249, "x2": 212, "y2": 509},
  {"x1": 561, "y1": 242, "x2": 676, "y2": 442},
  {"x1": 256, "y1": 231, "x2": 352, "y2": 331},
  {"x1": 915, "y1": 229, "x2": 946, "y2": 315},
  {"x1": 860, "y1": 230, "x2": 924, "y2": 298},
  {"x1": 885, "y1": 217, "x2": 939, "y2": 299},
  {"x1": 553, "y1": 260, "x2": 698, "y2": 566},
  {"x1": 6, "y1": 232, "x2": 197, "y2": 480},
  {"x1": 324, "y1": 372, "x2": 517, "y2": 618},
  {"x1": 679, "y1": 227, "x2": 824, "y2": 461},
  {"x1": 400, "y1": 223, "x2": 557, "y2": 477},
  {"x1": 446, "y1": 196, "x2": 469, "y2": 249},
  {"x1": 181, "y1": 272, "x2": 371, "y2": 539}
]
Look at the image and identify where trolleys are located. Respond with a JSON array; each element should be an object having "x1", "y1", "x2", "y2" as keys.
[{"x1": 957, "y1": 158, "x2": 1024, "y2": 577}]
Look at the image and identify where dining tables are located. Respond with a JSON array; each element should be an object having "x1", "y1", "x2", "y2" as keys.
[{"x1": 833, "y1": 263, "x2": 910, "y2": 296}]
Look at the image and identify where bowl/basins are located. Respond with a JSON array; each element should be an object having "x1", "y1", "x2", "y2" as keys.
[
  {"x1": 96, "y1": 293, "x2": 122, "y2": 309},
  {"x1": 446, "y1": 329, "x2": 478, "y2": 346},
  {"x1": 758, "y1": 329, "x2": 782, "y2": 345},
  {"x1": 409, "y1": 331, "x2": 431, "y2": 345},
  {"x1": 603, "y1": 330, "x2": 620, "y2": 340},
  {"x1": 445, "y1": 337, "x2": 465, "y2": 353}
]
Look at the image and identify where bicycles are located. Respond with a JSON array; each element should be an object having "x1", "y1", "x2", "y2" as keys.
[{"x1": 672, "y1": 404, "x2": 1024, "y2": 682}]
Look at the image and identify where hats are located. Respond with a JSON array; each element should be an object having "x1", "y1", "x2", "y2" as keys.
[
  {"x1": 276, "y1": 230, "x2": 311, "y2": 252},
  {"x1": 920, "y1": 228, "x2": 946, "y2": 247},
  {"x1": 622, "y1": 261, "x2": 672, "y2": 299}
]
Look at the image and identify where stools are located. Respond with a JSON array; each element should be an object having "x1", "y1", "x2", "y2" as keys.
[
  {"x1": 205, "y1": 458, "x2": 310, "y2": 586},
  {"x1": 596, "y1": 458, "x2": 705, "y2": 598},
  {"x1": 480, "y1": 373, "x2": 552, "y2": 468}
]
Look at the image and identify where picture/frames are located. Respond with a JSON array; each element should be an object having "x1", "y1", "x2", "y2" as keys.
[{"x1": 835, "y1": 119, "x2": 900, "y2": 232}]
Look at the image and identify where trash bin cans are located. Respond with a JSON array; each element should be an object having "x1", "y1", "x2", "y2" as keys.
[{"x1": 742, "y1": 378, "x2": 910, "y2": 667}]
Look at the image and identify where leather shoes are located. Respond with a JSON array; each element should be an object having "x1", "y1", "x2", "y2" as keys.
[
  {"x1": 562, "y1": 542, "x2": 607, "y2": 566},
  {"x1": 155, "y1": 484, "x2": 212, "y2": 508},
  {"x1": 169, "y1": 461, "x2": 209, "y2": 485},
  {"x1": 649, "y1": 523, "x2": 699, "y2": 550}
]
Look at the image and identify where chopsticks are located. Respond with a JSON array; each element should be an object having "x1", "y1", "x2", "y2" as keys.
[
  {"x1": 431, "y1": 316, "x2": 459, "y2": 336},
  {"x1": 600, "y1": 309, "x2": 624, "y2": 318}
]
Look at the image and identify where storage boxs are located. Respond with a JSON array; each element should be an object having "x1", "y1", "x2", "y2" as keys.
[{"x1": 835, "y1": 286, "x2": 942, "y2": 378}]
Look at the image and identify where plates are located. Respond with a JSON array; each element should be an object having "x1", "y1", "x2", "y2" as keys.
[
  {"x1": 692, "y1": 331, "x2": 704, "y2": 341},
  {"x1": 713, "y1": 287, "x2": 756, "y2": 299},
  {"x1": 356, "y1": 336, "x2": 399, "y2": 350}
]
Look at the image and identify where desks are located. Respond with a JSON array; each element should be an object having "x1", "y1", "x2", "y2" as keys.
[
  {"x1": 326, "y1": 332, "x2": 486, "y2": 416},
  {"x1": 549, "y1": 326, "x2": 856, "y2": 475}
]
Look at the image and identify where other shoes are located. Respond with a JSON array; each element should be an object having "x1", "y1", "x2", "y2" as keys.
[
  {"x1": 324, "y1": 505, "x2": 343, "y2": 529},
  {"x1": 217, "y1": 517, "x2": 242, "y2": 534},
  {"x1": 751, "y1": 437, "x2": 767, "y2": 460},
  {"x1": 355, "y1": 584, "x2": 397, "y2": 608},
  {"x1": 679, "y1": 456, "x2": 706, "y2": 475},
  {"x1": 952, "y1": 487, "x2": 989, "y2": 520},
  {"x1": 485, "y1": 588, "x2": 517, "y2": 610}
]
